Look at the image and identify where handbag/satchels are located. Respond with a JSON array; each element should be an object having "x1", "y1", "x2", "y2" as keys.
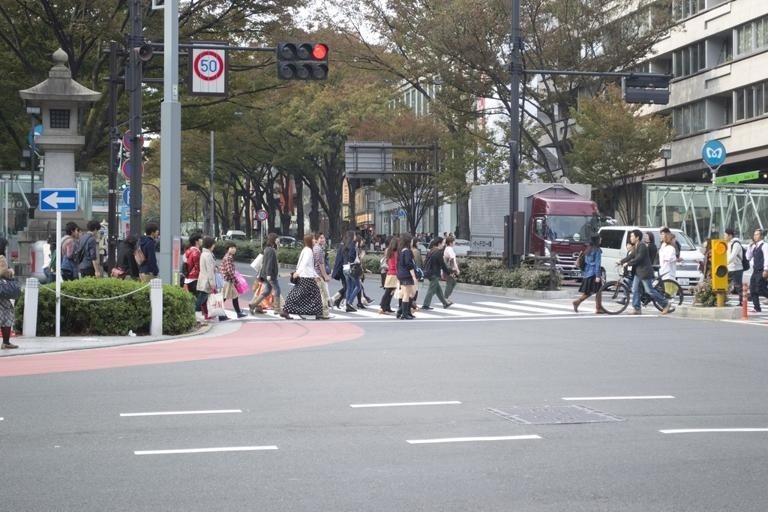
[
  {"x1": 134, "y1": 247, "x2": 146, "y2": 266},
  {"x1": 110, "y1": 268, "x2": 126, "y2": 279},
  {"x1": 290, "y1": 272, "x2": 301, "y2": 284},
  {"x1": 0, "y1": 283, "x2": 19, "y2": 299},
  {"x1": 250, "y1": 253, "x2": 265, "y2": 282},
  {"x1": 351, "y1": 263, "x2": 362, "y2": 277},
  {"x1": 182, "y1": 263, "x2": 189, "y2": 277},
  {"x1": 215, "y1": 272, "x2": 249, "y2": 300},
  {"x1": 384, "y1": 275, "x2": 400, "y2": 288},
  {"x1": 423, "y1": 253, "x2": 433, "y2": 278},
  {"x1": 414, "y1": 268, "x2": 424, "y2": 281},
  {"x1": 72, "y1": 248, "x2": 85, "y2": 264},
  {"x1": 575, "y1": 250, "x2": 586, "y2": 272}
]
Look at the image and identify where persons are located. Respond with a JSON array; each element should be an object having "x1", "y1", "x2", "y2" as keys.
[
  {"x1": 692, "y1": 229, "x2": 768, "y2": 313},
  {"x1": 618, "y1": 224, "x2": 681, "y2": 315},
  {"x1": 0, "y1": 255, "x2": 20, "y2": 349},
  {"x1": 0, "y1": 238, "x2": 13, "y2": 256},
  {"x1": 47, "y1": 219, "x2": 158, "y2": 284},
  {"x1": 179, "y1": 224, "x2": 461, "y2": 322},
  {"x1": 572, "y1": 233, "x2": 606, "y2": 314}
]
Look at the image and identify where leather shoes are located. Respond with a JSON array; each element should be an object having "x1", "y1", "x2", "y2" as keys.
[{"x1": 2, "y1": 343, "x2": 17, "y2": 349}]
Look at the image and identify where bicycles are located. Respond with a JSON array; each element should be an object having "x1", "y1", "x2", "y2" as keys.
[{"x1": 598, "y1": 261, "x2": 683, "y2": 314}]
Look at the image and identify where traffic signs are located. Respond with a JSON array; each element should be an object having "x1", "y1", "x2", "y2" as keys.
[{"x1": 193, "y1": 43, "x2": 225, "y2": 94}]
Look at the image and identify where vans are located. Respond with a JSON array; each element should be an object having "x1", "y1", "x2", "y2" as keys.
[
  {"x1": 595, "y1": 224, "x2": 704, "y2": 294},
  {"x1": 226, "y1": 229, "x2": 246, "y2": 236}
]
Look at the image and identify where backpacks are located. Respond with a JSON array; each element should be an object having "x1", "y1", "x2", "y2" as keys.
[{"x1": 730, "y1": 241, "x2": 750, "y2": 270}]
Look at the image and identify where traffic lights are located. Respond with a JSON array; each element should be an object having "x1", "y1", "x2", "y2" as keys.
[
  {"x1": 710, "y1": 238, "x2": 729, "y2": 292},
  {"x1": 276, "y1": 41, "x2": 329, "y2": 62},
  {"x1": 276, "y1": 60, "x2": 328, "y2": 80}
]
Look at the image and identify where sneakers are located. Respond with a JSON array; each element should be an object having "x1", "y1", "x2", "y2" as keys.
[
  {"x1": 300, "y1": 314, "x2": 306, "y2": 320},
  {"x1": 316, "y1": 314, "x2": 335, "y2": 319},
  {"x1": 237, "y1": 313, "x2": 247, "y2": 318},
  {"x1": 327, "y1": 298, "x2": 375, "y2": 312},
  {"x1": 596, "y1": 310, "x2": 606, "y2": 313},
  {"x1": 662, "y1": 301, "x2": 671, "y2": 313},
  {"x1": 573, "y1": 301, "x2": 577, "y2": 312},
  {"x1": 629, "y1": 308, "x2": 640, "y2": 314},
  {"x1": 250, "y1": 303, "x2": 292, "y2": 320},
  {"x1": 380, "y1": 298, "x2": 452, "y2": 320}
]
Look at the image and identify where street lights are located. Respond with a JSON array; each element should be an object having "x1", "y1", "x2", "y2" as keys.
[{"x1": 659, "y1": 148, "x2": 671, "y2": 180}]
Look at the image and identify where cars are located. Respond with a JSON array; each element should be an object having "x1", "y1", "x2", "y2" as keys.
[
  {"x1": 454, "y1": 239, "x2": 470, "y2": 265},
  {"x1": 731, "y1": 228, "x2": 768, "y2": 295},
  {"x1": 273, "y1": 236, "x2": 303, "y2": 250},
  {"x1": 415, "y1": 242, "x2": 431, "y2": 269}
]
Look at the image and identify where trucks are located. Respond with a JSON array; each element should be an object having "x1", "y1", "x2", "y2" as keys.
[{"x1": 467, "y1": 180, "x2": 609, "y2": 281}]
